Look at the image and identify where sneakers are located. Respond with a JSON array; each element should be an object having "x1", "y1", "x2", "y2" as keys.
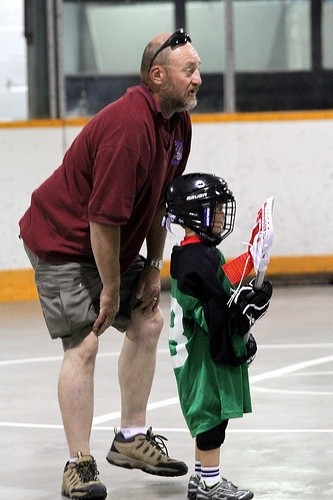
[
  {"x1": 187, "y1": 474, "x2": 201, "y2": 500},
  {"x1": 106, "y1": 425, "x2": 188, "y2": 477},
  {"x1": 61, "y1": 452, "x2": 107, "y2": 500},
  {"x1": 196, "y1": 477, "x2": 254, "y2": 500}
]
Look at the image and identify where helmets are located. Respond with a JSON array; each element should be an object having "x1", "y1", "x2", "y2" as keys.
[{"x1": 166, "y1": 172, "x2": 234, "y2": 247}]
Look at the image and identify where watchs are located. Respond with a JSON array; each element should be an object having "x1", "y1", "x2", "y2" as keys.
[{"x1": 145, "y1": 256, "x2": 166, "y2": 271}]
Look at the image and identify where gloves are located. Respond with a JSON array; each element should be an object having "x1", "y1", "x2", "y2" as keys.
[{"x1": 239, "y1": 278, "x2": 273, "y2": 328}]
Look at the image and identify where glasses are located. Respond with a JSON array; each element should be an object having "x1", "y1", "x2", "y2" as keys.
[{"x1": 148, "y1": 28, "x2": 192, "y2": 72}]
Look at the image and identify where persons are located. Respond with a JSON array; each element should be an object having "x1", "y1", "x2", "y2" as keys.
[
  {"x1": 19, "y1": 32, "x2": 202, "y2": 499},
  {"x1": 168, "y1": 173, "x2": 273, "y2": 500}
]
